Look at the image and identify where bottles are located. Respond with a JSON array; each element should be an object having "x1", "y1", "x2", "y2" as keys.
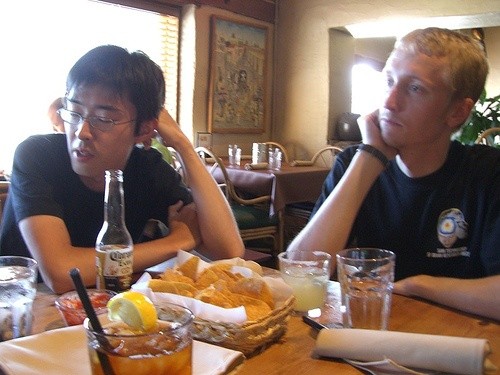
[{"x1": 95, "y1": 169, "x2": 134, "y2": 294}]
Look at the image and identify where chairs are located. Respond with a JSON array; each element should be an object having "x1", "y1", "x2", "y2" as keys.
[{"x1": 195, "y1": 140, "x2": 344, "y2": 270}]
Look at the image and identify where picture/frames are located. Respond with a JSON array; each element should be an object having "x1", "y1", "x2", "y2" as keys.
[{"x1": 208, "y1": 14, "x2": 273, "y2": 134}]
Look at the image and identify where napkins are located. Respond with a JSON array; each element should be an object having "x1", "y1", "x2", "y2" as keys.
[{"x1": 312, "y1": 327, "x2": 497, "y2": 375}]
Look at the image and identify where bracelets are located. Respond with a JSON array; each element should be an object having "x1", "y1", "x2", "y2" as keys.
[{"x1": 357, "y1": 145, "x2": 388, "y2": 165}]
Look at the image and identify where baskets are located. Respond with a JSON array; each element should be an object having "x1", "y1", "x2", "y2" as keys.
[{"x1": 192, "y1": 295, "x2": 296, "y2": 356}]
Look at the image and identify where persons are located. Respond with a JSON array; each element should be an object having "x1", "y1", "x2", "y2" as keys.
[
  {"x1": 0, "y1": 45, "x2": 245, "y2": 294},
  {"x1": 287, "y1": 26, "x2": 500, "y2": 322}
]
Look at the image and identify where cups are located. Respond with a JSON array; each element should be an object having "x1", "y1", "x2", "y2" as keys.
[
  {"x1": 228, "y1": 145, "x2": 242, "y2": 166},
  {"x1": 252, "y1": 142, "x2": 266, "y2": 163},
  {"x1": 54, "y1": 290, "x2": 118, "y2": 326},
  {"x1": 0, "y1": 256, "x2": 38, "y2": 342},
  {"x1": 83, "y1": 302, "x2": 194, "y2": 375},
  {"x1": 269, "y1": 148, "x2": 281, "y2": 171},
  {"x1": 336, "y1": 248, "x2": 395, "y2": 330},
  {"x1": 277, "y1": 250, "x2": 331, "y2": 312}
]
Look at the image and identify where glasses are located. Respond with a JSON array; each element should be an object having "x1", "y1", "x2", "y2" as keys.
[{"x1": 57, "y1": 107, "x2": 139, "y2": 131}]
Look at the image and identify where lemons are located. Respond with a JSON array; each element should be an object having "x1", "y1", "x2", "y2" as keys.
[{"x1": 107, "y1": 291, "x2": 158, "y2": 333}]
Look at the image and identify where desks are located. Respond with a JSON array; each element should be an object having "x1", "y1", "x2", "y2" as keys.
[
  {"x1": 0, "y1": 258, "x2": 500, "y2": 375},
  {"x1": 205, "y1": 158, "x2": 331, "y2": 250}
]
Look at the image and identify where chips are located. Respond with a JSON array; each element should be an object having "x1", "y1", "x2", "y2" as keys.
[{"x1": 148, "y1": 257, "x2": 274, "y2": 321}]
